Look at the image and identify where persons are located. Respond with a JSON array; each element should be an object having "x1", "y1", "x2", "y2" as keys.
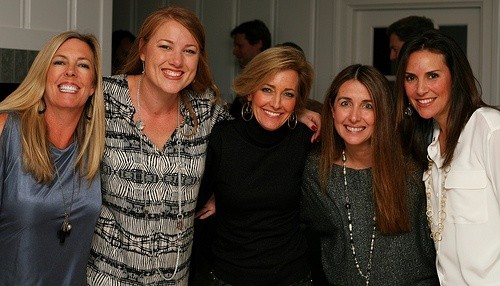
[
  {"x1": 112, "y1": 30, "x2": 137, "y2": 65},
  {"x1": 396, "y1": 29, "x2": 500, "y2": 286},
  {"x1": 230, "y1": 19, "x2": 272, "y2": 68},
  {"x1": 194, "y1": 63, "x2": 441, "y2": 286},
  {"x1": 341, "y1": 145, "x2": 376, "y2": 286},
  {"x1": 0, "y1": 31, "x2": 108, "y2": 286},
  {"x1": 194, "y1": 46, "x2": 317, "y2": 286},
  {"x1": 87, "y1": 5, "x2": 323, "y2": 286},
  {"x1": 389, "y1": 16, "x2": 435, "y2": 60}
]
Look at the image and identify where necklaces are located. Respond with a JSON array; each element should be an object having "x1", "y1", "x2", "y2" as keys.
[
  {"x1": 426, "y1": 136, "x2": 451, "y2": 240},
  {"x1": 138, "y1": 72, "x2": 183, "y2": 280},
  {"x1": 48, "y1": 131, "x2": 78, "y2": 233}
]
